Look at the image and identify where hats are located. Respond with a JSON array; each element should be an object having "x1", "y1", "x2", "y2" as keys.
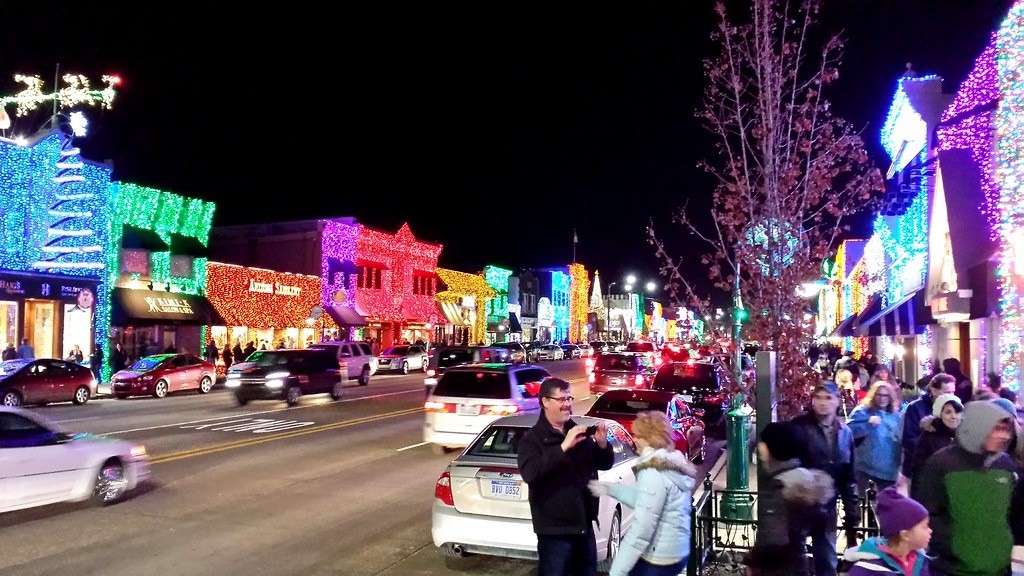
[
  {"x1": 813, "y1": 379, "x2": 837, "y2": 393},
  {"x1": 932, "y1": 393, "x2": 964, "y2": 419},
  {"x1": 875, "y1": 487, "x2": 929, "y2": 537}
]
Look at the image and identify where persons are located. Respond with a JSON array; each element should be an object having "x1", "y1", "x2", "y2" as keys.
[
  {"x1": 364, "y1": 336, "x2": 380, "y2": 356},
  {"x1": 110, "y1": 339, "x2": 178, "y2": 377},
  {"x1": 589, "y1": 410, "x2": 696, "y2": 576},
  {"x1": 91, "y1": 344, "x2": 104, "y2": 384},
  {"x1": 206, "y1": 340, "x2": 286, "y2": 376},
  {"x1": 518, "y1": 378, "x2": 614, "y2": 576},
  {"x1": 394, "y1": 337, "x2": 484, "y2": 346},
  {"x1": 741, "y1": 338, "x2": 1024, "y2": 576},
  {"x1": 2, "y1": 337, "x2": 34, "y2": 362},
  {"x1": 69, "y1": 345, "x2": 84, "y2": 364}
]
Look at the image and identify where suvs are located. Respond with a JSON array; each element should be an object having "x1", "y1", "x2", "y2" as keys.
[
  {"x1": 306, "y1": 341, "x2": 374, "y2": 386},
  {"x1": 225, "y1": 348, "x2": 343, "y2": 405},
  {"x1": 522, "y1": 342, "x2": 540, "y2": 362},
  {"x1": 425, "y1": 346, "x2": 502, "y2": 396}
]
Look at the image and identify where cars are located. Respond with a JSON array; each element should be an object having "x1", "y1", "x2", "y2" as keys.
[
  {"x1": 539, "y1": 345, "x2": 564, "y2": 361},
  {"x1": 560, "y1": 344, "x2": 583, "y2": 359},
  {"x1": 590, "y1": 350, "x2": 659, "y2": 399},
  {"x1": 430, "y1": 413, "x2": 640, "y2": 573},
  {"x1": 108, "y1": 353, "x2": 219, "y2": 400},
  {"x1": 579, "y1": 344, "x2": 594, "y2": 357},
  {"x1": 423, "y1": 362, "x2": 557, "y2": 453},
  {"x1": 0, "y1": 406, "x2": 149, "y2": 517},
  {"x1": 374, "y1": 343, "x2": 429, "y2": 373},
  {"x1": 0, "y1": 358, "x2": 97, "y2": 407},
  {"x1": 589, "y1": 340, "x2": 626, "y2": 355},
  {"x1": 627, "y1": 340, "x2": 756, "y2": 392},
  {"x1": 491, "y1": 341, "x2": 526, "y2": 362},
  {"x1": 585, "y1": 388, "x2": 706, "y2": 466},
  {"x1": 650, "y1": 362, "x2": 732, "y2": 432}
]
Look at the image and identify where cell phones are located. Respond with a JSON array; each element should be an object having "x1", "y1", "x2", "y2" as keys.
[{"x1": 582, "y1": 426, "x2": 598, "y2": 436}]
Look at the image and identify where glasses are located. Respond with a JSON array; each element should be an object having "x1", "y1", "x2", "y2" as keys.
[{"x1": 545, "y1": 396, "x2": 574, "y2": 403}]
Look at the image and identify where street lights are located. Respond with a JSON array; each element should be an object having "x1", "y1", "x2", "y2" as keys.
[
  {"x1": 630, "y1": 282, "x2": 655, "y2": 339},
  {"x1": 607, "y1": 275, "x2": 636, "y2": 341},
  {"x1": 719, "y1": 216, "x2": 798, "y2": 521}
]
